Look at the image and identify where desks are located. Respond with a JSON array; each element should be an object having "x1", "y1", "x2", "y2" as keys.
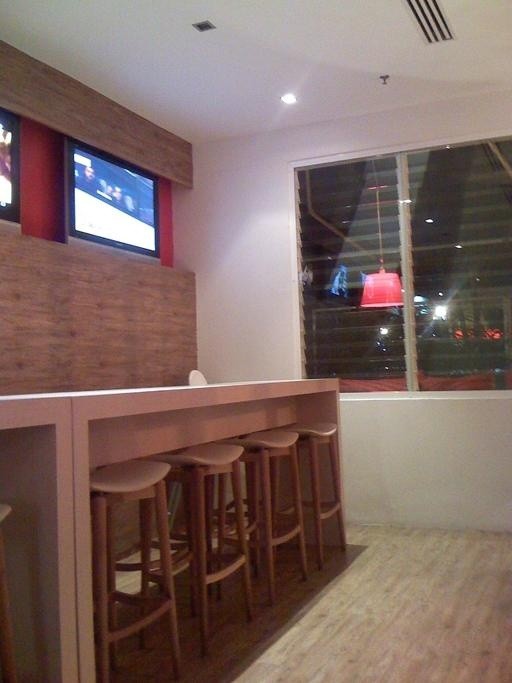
[
  {"x1": 1, "y1": 393, "x2": 79, "y2": 683},
  {"x1": 72, "y1": 377, "x2": 345, "y2": 683}
]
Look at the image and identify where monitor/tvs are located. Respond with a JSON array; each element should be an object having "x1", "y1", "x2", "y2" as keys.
[
  {"x1": 0, "y1": 106, "x2": 21, "y2": 235},
  {"x1": 64, "y1": 139, "x2": 161, "y2": 267}
]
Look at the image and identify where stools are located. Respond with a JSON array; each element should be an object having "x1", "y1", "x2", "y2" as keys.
[
  {"x1": 89, "y1": 460, "x2": 185, "y2": 683},
  {"x1": 154, "y1": 446, "x2": 253, "y2": 656},
  {"x1": 0, "y1": 504, "x2": 17, "y2": 683},
  {"x1": 215, "y1": 430, "x2": 309, "y2": 607},
  {"x1": 285, "y1": 422, "x2": 346, "y2": 570}
]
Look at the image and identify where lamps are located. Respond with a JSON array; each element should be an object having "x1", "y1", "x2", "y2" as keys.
[{"x1": 359, "y1": 186, "x2": 404, "y2": 307}]
